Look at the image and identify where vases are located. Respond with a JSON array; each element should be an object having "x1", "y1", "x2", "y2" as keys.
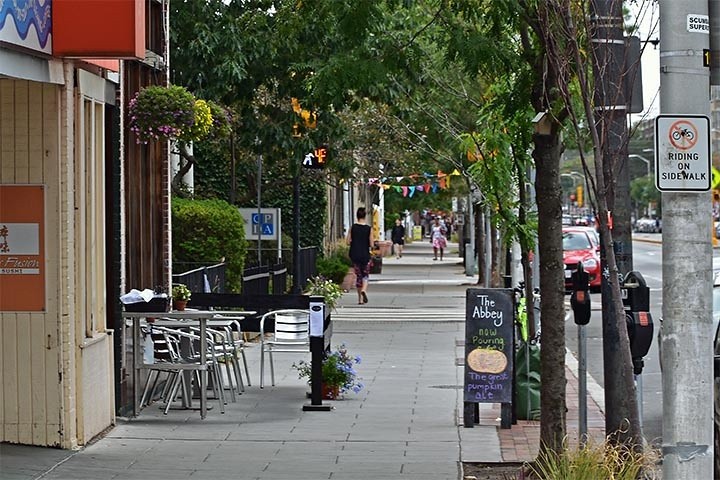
[{"x1": 322, "y1": 383, "x2": 340, "y2": 400}]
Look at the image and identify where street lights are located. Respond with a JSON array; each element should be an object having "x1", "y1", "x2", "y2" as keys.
[
  {"x1": 628, "y1": 154, "x2": 652, "y2": 217},
  {"x1": 560, "y1": 173, "x2": 576, "y2": 188},
  {"x1": 571, "y1": 172, "x2": 588, "y2": 216}
]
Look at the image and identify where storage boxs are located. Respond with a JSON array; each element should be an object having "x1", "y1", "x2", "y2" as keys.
[{"x1": 124, "y1": 296, "x2": 171, "y2": 313}]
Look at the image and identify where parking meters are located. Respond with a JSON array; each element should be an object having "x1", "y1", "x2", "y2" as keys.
[
  {"x1": 618, "y1": 272, "x2": 653, "y2": 440},
  {"x1": 570, "y1": 262, "x2": 593, "y2": 449}
]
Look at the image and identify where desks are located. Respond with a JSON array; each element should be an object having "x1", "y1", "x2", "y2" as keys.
[
  {"x1": 124, "y1": 312, "x2": 215, "y2": 419},
  {"x1": 196, "y1": 311, "x2": 258, "y2": 386}
]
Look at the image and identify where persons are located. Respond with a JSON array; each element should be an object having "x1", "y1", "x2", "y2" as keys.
[
  {"x1": 391, "y1": 218, "x2": 405, "y2": 259},
  {"x1": 430, "y1": 218, "x2": 446, "y2": 261},
  {"x1": 445, "y1": 219, "x2": 453, "y2": 241},
  {"x1": 431, "y1": 217, "x2": 447, "y2": 232},
  {"x1": 346, "y1": 207, "x2": 374, "y2": 305}
]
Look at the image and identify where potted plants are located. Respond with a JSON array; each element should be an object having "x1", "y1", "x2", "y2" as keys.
[
  {"x1": 172, "y1": 285, "x2": 191, "y2": 311},
  {"x1": 316, "y1": 246, "x2": 357, "y2": 291}
]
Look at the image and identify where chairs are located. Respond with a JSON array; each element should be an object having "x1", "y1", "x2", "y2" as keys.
[{"x1": 138, "y1": 308, "x2": 315, "y2": 415}]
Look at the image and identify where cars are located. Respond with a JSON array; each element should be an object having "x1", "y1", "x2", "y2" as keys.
[
  {"x1": 561, "y1": 214, "x2": 590, "y2": 226},
  {"x1": 560, "y1": 226, "x2": 601, "y2": 294},
  {"x1": 656, "y1": 257, "x2": 720, "y2": 479}
]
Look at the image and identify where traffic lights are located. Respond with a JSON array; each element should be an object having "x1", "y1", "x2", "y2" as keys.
[
  {"x1": 301, "y1": 146, "x2": 331, "y2": 170},
  {"x1": 577, "y1": 186, "x2": 585, "y2": 207},
  {"x1": 291, "y1": 71, "x2": 319, "y2": 136}
]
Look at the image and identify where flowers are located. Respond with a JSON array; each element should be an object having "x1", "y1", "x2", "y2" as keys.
[
  {"x1": 177, "y1": 100, "x2": 211, "y2": 142},
  {"x1": 292, "y1": 342, "x2": 366, "y2": 400},
  {"x1": 209, "y1": 103, "x2": 231, "y2": 140},
  {"x1": 128, "y1": 86, "x2": 196, "y2": 144},
  {"x1": 307, "y1": 274, "x2": 345, "y2": 312}
]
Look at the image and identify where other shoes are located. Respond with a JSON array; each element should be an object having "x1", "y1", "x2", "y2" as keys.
[
  {"x1": 433, "y1": 258, "x2": 437, "y2": 260},
  {"x1": 359, "y1": 302, "x2": 364, "y2": 305},
  {"x1": 440, "y1": 256, "x2": 443, "y2": 261},
  {"x1": 361, "y1": 291, "x2": 368, "y2": 303},
  {"x1": 396, "y1": 254, "x2": 401, "y2": 259}
]
